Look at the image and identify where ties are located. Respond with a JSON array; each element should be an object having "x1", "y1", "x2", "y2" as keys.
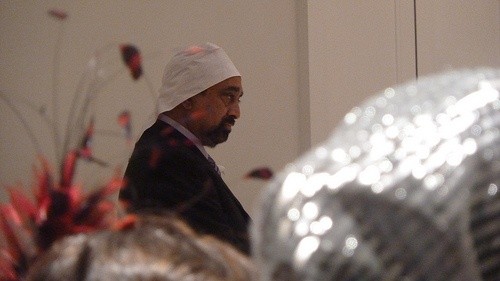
[{"x1": 207, "y1": 154, "x2": 221, "y2": 178}]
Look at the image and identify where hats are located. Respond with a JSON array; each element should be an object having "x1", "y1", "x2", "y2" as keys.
[{"x1": 154, "y1": 42, "x2": 241, "y2": 116}]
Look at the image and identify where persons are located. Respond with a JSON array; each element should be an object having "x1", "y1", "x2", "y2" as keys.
[
  {"x1": 116, "y1": 41, "x2": 255, "y2": 258},
  {"x1": 22, "y1": 223, "x2": 263, "y2": 281}
]
